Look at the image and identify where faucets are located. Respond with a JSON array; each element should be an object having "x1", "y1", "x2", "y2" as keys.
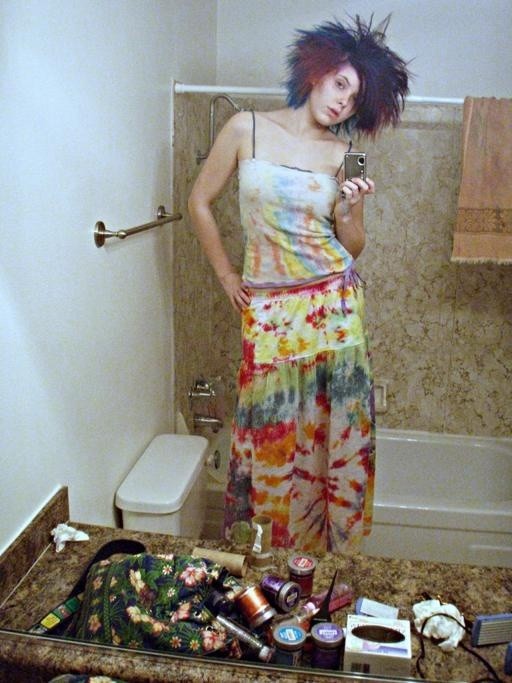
[{"x1": 196, "y1": 414, "x2": 223, "y2": 433}]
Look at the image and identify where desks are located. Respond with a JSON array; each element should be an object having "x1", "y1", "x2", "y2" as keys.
[{"x1": 0, "y1": 485, "x2": 512, "y2": 683}]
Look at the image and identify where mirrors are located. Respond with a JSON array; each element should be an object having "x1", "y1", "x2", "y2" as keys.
[{"x1": 0, "y1": 0, "x2": 512, "y2": 683}]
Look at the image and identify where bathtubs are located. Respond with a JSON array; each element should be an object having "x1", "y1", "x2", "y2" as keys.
[{"x1": 203, "y1": 417, "x2": 511, "y2": 568}]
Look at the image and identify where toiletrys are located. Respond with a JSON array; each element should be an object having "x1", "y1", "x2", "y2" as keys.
[{"x1": 202, "y1": 550, "x2": 354, "y2": 670}]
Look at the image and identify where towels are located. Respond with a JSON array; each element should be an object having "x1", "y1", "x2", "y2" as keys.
[{"x1": 449, "y1": 95, "x2": 512, "y2": 266}]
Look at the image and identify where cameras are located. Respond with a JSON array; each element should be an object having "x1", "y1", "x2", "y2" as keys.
[{"x1": 344, "y1": 152, "x2": 366, "y2": 192}]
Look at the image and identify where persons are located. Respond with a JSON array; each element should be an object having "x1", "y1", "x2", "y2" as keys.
[{"x1": 185, "y1": 10, "x2": 406, "y2": 555}]
